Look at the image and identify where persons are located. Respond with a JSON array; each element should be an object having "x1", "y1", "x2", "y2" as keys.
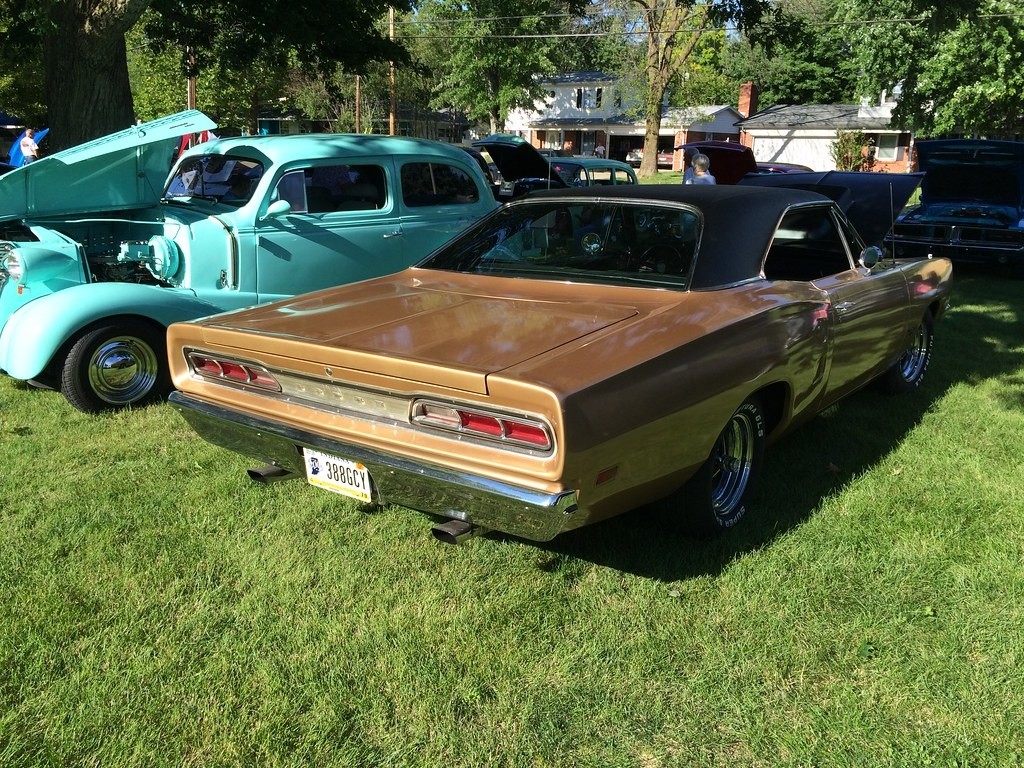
[
  {"x1": 20, "y1": 129, "x2": 40, "y2": 165},
  {"x1": 681, "y1": 147, "x2": 717, "y2": 186},
  {"x1": 595, "y1": 142, "x2": 605, "y2": 158},
  {"x1": 566, "y1": 140, "x2": 576, "y2": 150}
]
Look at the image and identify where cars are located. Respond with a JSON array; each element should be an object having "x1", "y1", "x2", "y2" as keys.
[
  {"x1": 486, "y1": 109, "x2": 1024, "y2": 296},
  {"x1": 0, "y1": 107, "x2": 575, "y2": 413},
  {"x1": 166, "y1": 184, "x2": 954, "y2": 544}
]
